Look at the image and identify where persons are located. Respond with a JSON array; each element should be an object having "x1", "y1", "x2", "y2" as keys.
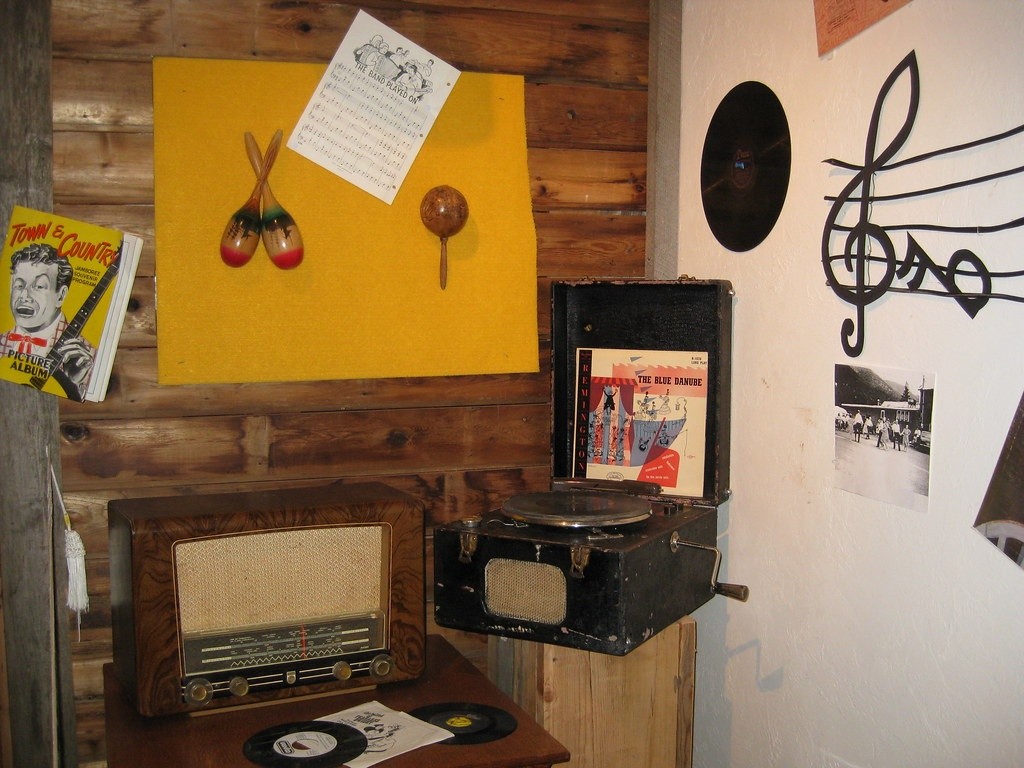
[{"x1": 839, "y1": 407, "x2": 922, "y2": 452}]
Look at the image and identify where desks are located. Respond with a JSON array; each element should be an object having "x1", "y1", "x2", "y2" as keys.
[{"x1": 101, "y1": 631, "x2": 572, "y2": 768}]
